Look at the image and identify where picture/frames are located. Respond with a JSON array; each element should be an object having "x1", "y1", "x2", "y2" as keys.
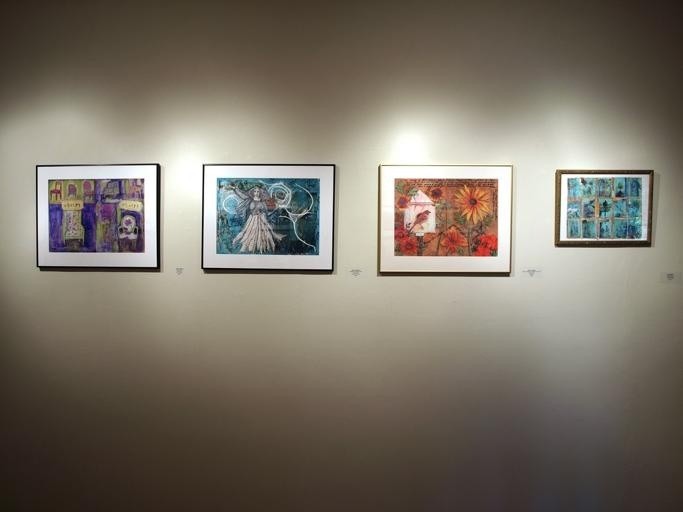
[
  {"x1": 36, "y1": 164, "x2": 161, "y2": 272},
  {"x1": 378, "y1": 164, "x2": 513, "y2": 276},
  {"x1": 201, "y1": 163, "x2": 335, "y2": 274},
  {"x1": 555, "y1": 169, "x2": 654, "y2": 247}
]
